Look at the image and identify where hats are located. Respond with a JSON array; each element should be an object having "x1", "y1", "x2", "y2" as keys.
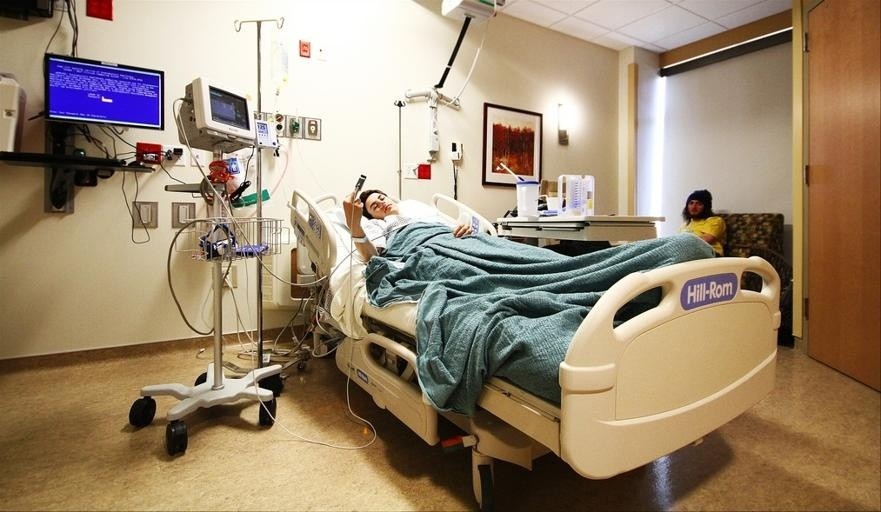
[{"x1": 686, "y1": 189, "x2": 713, "y2": 210}]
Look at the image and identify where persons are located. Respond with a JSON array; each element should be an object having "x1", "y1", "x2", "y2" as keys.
[
  {"x1": 675, "y1": 189, "x2": 728, "y2": 257},
  {"x1": 342, "y1": 188, "x2": 476, "y2": 260}
]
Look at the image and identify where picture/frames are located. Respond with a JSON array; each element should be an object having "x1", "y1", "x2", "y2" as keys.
[{"x1": 481, "y1": 102, "x2": 543, "y2": 189}]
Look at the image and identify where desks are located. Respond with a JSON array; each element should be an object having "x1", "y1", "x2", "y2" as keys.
[{"x1": 497, "y1": 213, "x2": 666, "y2": 247}]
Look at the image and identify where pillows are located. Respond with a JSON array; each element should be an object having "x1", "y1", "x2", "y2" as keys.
[{"x1": 327, "y1": 200, "x2": 461, "y2": 265}]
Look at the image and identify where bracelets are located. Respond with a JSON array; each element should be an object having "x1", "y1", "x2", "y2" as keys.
[{"x1": 352, "y1": 235, "x2": 368, "y2": 243}]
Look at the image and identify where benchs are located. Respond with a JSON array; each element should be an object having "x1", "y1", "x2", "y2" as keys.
[{"x1": 711, "y1": 211, "x2": 786, "y2": 293}]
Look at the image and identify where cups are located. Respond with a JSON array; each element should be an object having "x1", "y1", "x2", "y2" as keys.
[{"x1": 546, "y1": 197, "x2": 564, "y2": 211}]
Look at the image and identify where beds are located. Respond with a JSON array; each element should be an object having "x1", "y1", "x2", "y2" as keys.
[{"x1": 289, "y1": 188, "x2": 782, "y2": 512}]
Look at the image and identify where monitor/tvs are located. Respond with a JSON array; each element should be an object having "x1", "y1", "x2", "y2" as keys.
[
  {"x1": 44, "y1": 52, "x2": 165, "y2": 130},
  {"x1": 177, "y1": 77, "x2": 256, "y2": 153}
]
